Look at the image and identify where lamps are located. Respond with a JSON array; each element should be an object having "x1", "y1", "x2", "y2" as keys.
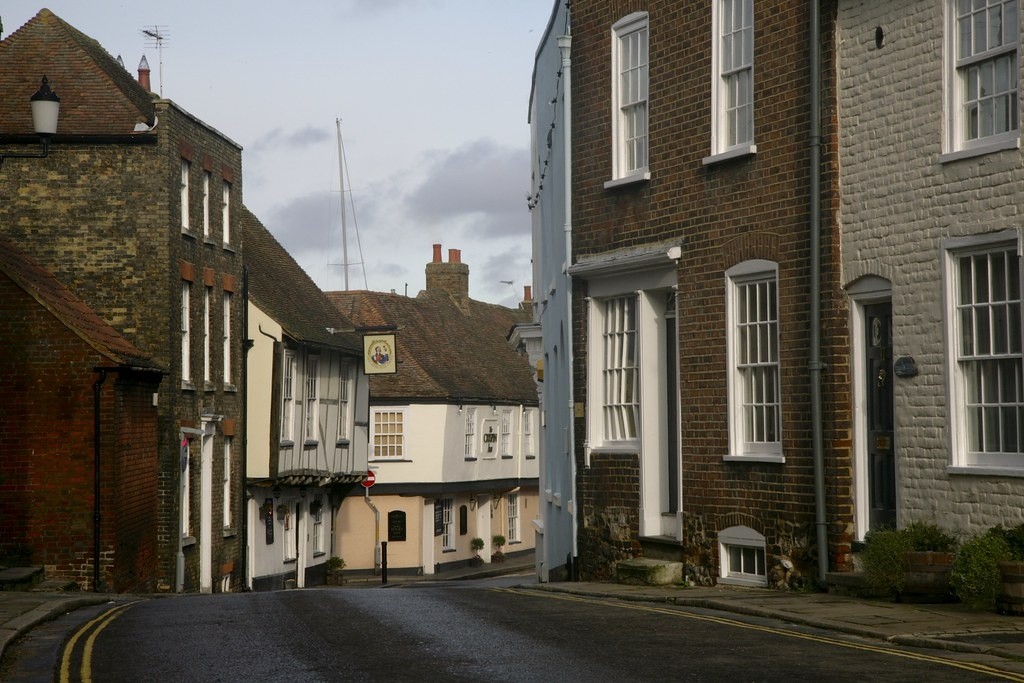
[{"x1": 0, "y1": 74, "x2": 61, "y2": 171}]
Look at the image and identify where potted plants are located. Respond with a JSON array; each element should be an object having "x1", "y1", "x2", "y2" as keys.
[
  {"x1": 948, "y1": 524, "x2": 1024, "y2": 615},
  {"x1": 470, "y1": 537, "x2": 484, "y2": 568},
  {"x1": 326, "y1": 557, "x2": 344, "y2": 585},
  {"x1": 492, "y1": 535, "x2": 506, "y2": 564},
  {"x1": 864, "y1": 523, "x2": 957, "y2": 602}
]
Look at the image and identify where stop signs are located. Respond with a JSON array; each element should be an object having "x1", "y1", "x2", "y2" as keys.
[{"x1": 361, "y1": 470, "x2": 376, "y2": 488}]
